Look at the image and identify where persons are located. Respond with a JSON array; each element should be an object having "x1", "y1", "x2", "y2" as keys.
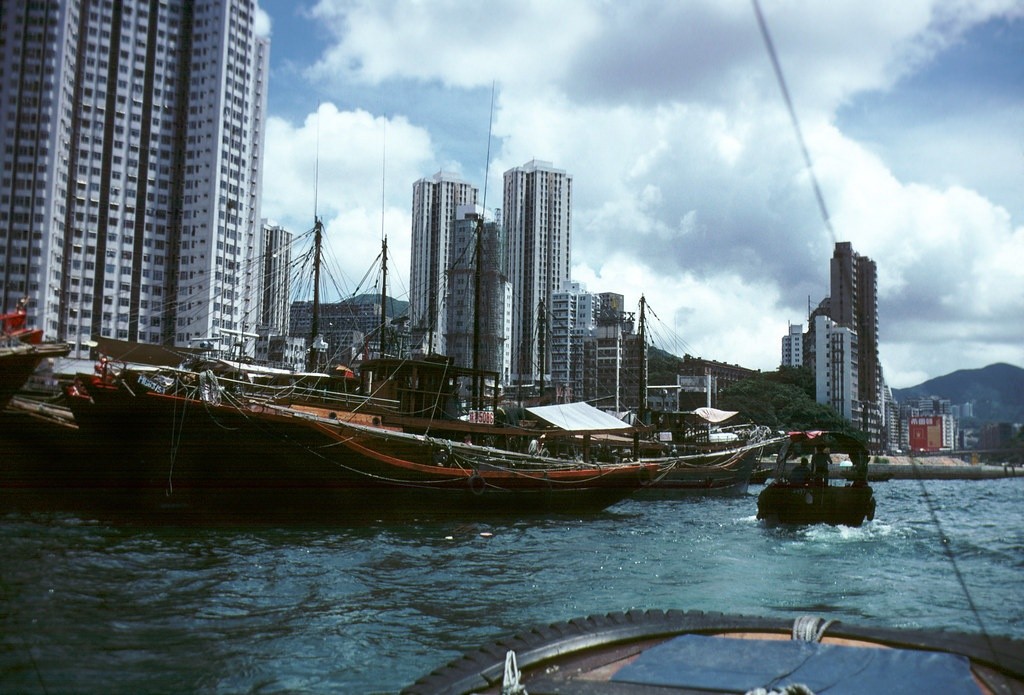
[
  {"x1": 811, "y1": 445, "x2": 833, "y2": 486},
  {"x1": 789, "y1": 458, "x2": 811, "y2": 486}
]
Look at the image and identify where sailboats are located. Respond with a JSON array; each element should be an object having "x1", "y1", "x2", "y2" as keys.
[{"x1": 0, "y1": 218, "x2": 768, "y2": 508}]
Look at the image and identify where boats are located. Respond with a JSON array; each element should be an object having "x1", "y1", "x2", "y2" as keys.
[{"x1": 756, "y1": 429, "x2": 877, "y2": 527}]
